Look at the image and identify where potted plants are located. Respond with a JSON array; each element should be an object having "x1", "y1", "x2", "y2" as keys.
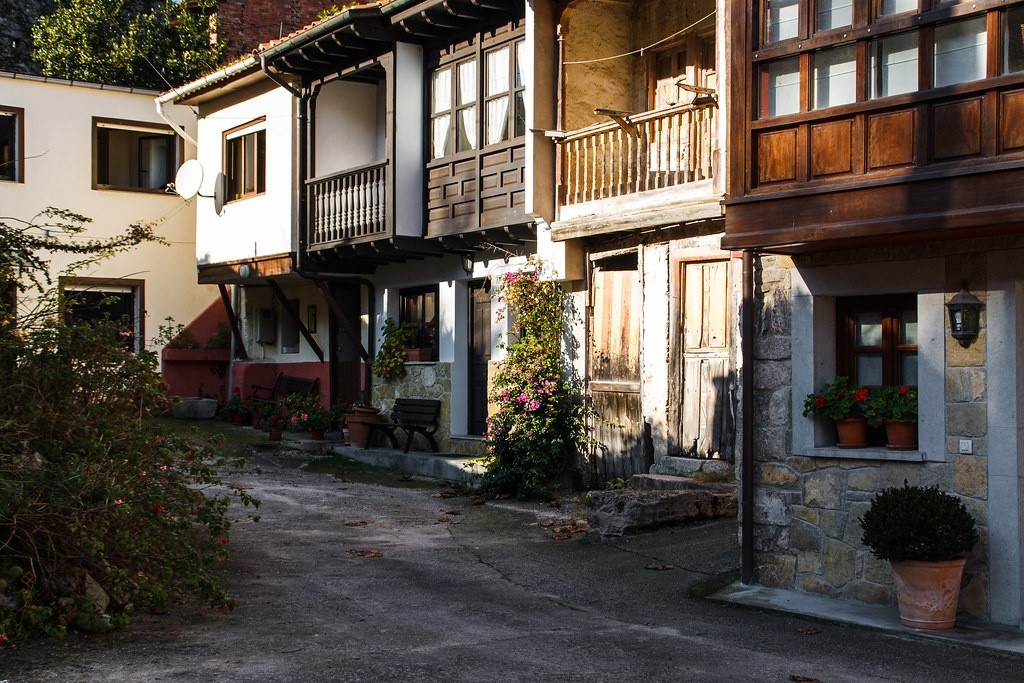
[{"x1": 856, "y1": 478, "x2": 981, "y2": 631}]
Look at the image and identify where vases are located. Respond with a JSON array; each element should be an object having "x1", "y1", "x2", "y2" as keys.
[
  {"x1": 311, "y1": 428, "x2": 325, "y2": 441},
  {"x1": 835, "y1": 418, "x2": 870, "y2": 449},
  {"x1": 405, "y1": 348, "x2": 432, "y2": 361},
  {"x1": 342, "y1": 414, "x2": 384, "y2": 448},
  {"x1": 232, "y1": 413, "x2": 246, "y2": 426},
  {"x1": 269, "y1": 427, "x2": 282, "y2": 441},
  {"x1": 252, "y1": 415, "x2": 262, "y2": 429},
  {"x1": 169, "y1": 396, "x2": 217, "y2": 419},
  {"x1": 339, "y1": 423, "x2": 347, "y2": 440},
  {"x1": 352, "y1": 406, "x2": 381, "y2": 415},
  {"x1": 884, "y1": 420, "x2": 918, "y2": 451}
]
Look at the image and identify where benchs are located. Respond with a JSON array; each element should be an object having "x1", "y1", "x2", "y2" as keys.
[
  {"x1": 361, "y1": 398, "x2": 441, "y2": 455},
  {"x1": 251, "y1": 372, "x2": 319, "y2": 428}
]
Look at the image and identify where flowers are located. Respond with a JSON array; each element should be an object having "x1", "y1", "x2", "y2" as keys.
[
  {"x1": 801, "y1": 375, "x2": 870, "y2": 420},
  {"x1": 219, "y1": 387, "x2": 248, "y2": 416},
  {"x1": 370, "y1": 315, "x2": 433, "y2": 384},
  {"x1": 860, "y1": 384, "x2": 918, "y2": 429},
  {"x1": 286, "y1": 412, "x2": 329, "y2": 431},
  {"x1": 324, "y1": 395, "x2": 365, "y2": 422},
  {"x1": 245, "y1": 399, "x2": 273, "y2": 418},
  {"x1": 259, "y1": 409, "x2": 289, "y2": 434}
]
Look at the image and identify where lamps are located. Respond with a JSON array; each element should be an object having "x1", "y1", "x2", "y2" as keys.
[
  {"x1": 944, "y1": 280, "x2": 984, "y2": 349},
  {"x1": 460, "y1": 244, "x2": 474, "y2": 277}
]
